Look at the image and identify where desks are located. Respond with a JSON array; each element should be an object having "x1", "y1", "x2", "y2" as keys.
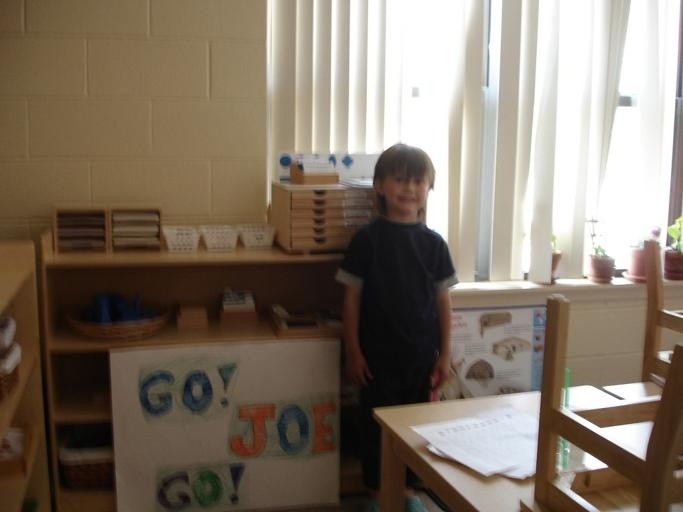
[{"x1": 371, "y1": 382, "x2": 679, "y2": 511}]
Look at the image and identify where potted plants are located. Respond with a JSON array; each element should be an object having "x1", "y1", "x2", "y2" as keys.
[{"x1": 551, "y1": 215, "x2": 682, "y2": 285}]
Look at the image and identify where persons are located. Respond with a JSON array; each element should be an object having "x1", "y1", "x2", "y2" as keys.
[{"x1": 335, "y1": 143, "x2": 459, "y2": 512}]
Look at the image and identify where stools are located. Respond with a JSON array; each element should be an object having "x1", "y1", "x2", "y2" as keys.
[{"x1": 519, "y1": 240, "x2": 683, "y2": 510}]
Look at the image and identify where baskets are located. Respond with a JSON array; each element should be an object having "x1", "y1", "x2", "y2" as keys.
[
  {"x1": 201, "y1": 223, "x2": 240, "y2": 249},
  {"x1": 61, "y1": 451, "x2": 115, "y2": 492},
  {"x1": 62, "y1": 295, "x2": 177, "y2": 342},
  {"x1": 239, "y1": 225, "x2": 276, "y2": 247},
  {"x1": 162, "y1": 225, "x2": 200, "y2": 251}
]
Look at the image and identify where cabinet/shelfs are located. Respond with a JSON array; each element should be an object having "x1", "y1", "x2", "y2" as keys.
[
  {"x1": 0, "y1": 241, "x2": 53, "y2": 512},
  {"x1": 40, "y1": 248, "x2": 369, "y2": 509}
]
